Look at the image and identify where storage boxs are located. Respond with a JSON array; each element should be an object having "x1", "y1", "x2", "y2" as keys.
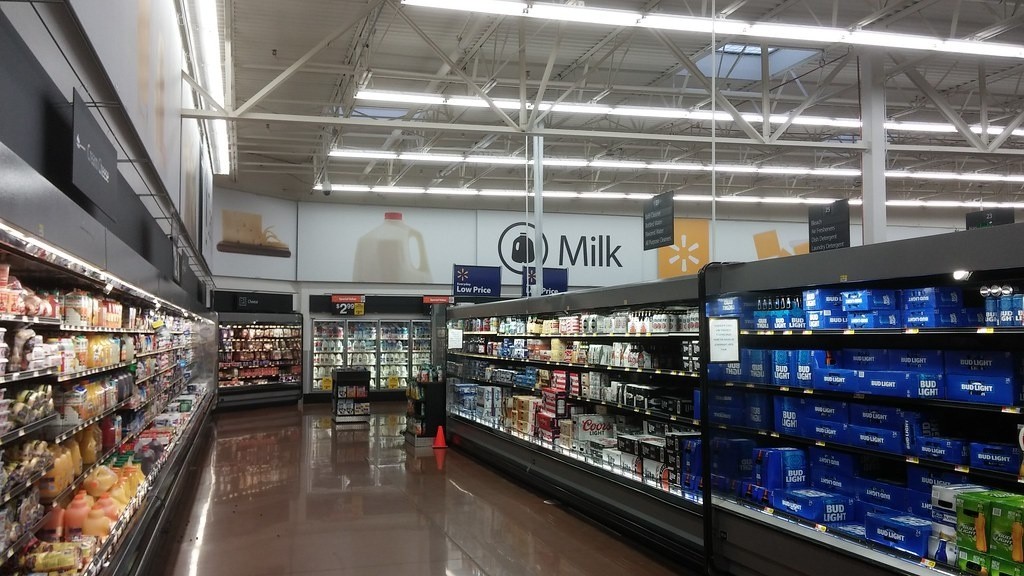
[
  {"x1": 956, "y1": 489, "x2": 1022, "y2": 554},
  {"x1": 956, "y1": 546, "x2": 991, "y2": 576},
  {"x1": 807, "y1": 446, "x2": 876, "y2": 476},
  {"x1": 932, "y1": 482, "x2": 993, "y2": 543},
  {"x1": 905, "y1": 488, "x2": 932, "y2": 518},
  {"x1": 853, "y1": 500, "x2": 898, "y2": 525},
  {"x1": 773, "y1": 488, "x2": 854, "y2": 525},
  {"x1": 753, "y1": 287, "x2": 985, "y2": 328},
  {"x1": 851, "y1": 474, "x2": 906, "y2": 512},
  {"x1": 914, "y1": 437, "x2": 1023, "y2": 474},
  {"x1": 907, "y1": 466, "x2": 979, "y2": 494},
  {"x1": 809, "y1": 465, "x2": 855, "y2": 498},
  {"x1": 928, "y1": 536, "x2": 957, "y2": 566},
  {"x1": 848, "y1": 424, "x2": 911, "y2": 454},
  {"x1": 990, "y1": 495, "x2": 1024, "y2": 564},
  {"x1": 742, "y1": 447, "x2": 809, "y2": 508},
  {"x1": 989, "y1": 555, "x2": 1024, "y2": 576},
  {"x1": 771, "y1": 349, "x2": 1024, "y2": 436},
  {"x1": 865, "y1": 511, "x2": 932, "y2": 557},
  {"x1": 452, "y1": 319, "x2": 703, "y2": 496}
]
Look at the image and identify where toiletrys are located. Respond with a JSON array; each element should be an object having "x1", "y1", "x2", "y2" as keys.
[{"x1": 444, "y1": 306, "x2": 698, "y2": 429}]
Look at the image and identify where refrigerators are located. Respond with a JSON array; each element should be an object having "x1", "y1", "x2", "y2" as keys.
[{"x1": 302, "y1": 319, "x2": 434, "y2": 404}]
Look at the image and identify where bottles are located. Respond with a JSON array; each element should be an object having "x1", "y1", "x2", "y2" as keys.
[{"x1": 352, "y1": 212, "x2": 433, "y2": 282}]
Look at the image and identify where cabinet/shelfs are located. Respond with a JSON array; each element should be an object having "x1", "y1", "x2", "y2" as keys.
[
  {"x1": 445, "y1": 274, "x2": 710, "y2": 576},
  {"x1": 330, "y1": 370, "x2": 371, "y2": 425},
  {"x1": 216, "y1": 311, "x2": 303, "y2": 412},
  {"x1": 0, "y1": 140, "x2": 216, "y2": 576},
  {"x1": 405, "y1": 377, "x2": 446, "y2": 438},
  {"x1": 705, "y1": 222, "x2": 1024, "y2": 576}
]
